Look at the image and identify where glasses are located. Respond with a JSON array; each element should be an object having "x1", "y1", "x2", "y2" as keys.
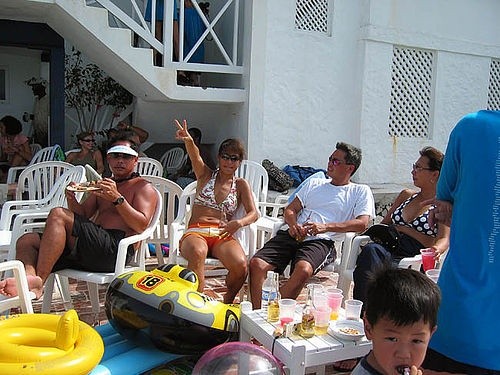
[
  {"x1": 412, "y1": 164, "x2": 434, "y2": 173},
  {"x1": 81, "y1": 139, "x2": 95, "y2": 142},
  {"x1": 220, "y1": 153, "x2": 243, "y2": 162},
  {"x1": 329, "y1": 155, "x2": 356, "y2": 165},
  {"x1": 110, "y1": 153, "x2": 135, "y2": 159}
]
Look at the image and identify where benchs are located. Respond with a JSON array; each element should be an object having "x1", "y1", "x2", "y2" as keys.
[{"x1": 267, "y1": 184, "x2": 408, "y2": 218}]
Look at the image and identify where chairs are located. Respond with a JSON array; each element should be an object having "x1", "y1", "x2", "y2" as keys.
[{"x1": 0, "y1": 144, "x2": 450, "y2": 314}]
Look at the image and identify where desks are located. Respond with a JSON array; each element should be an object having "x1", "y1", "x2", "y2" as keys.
[{"x1": 239, "y1": 301, "x2": 374, "y2": 375}]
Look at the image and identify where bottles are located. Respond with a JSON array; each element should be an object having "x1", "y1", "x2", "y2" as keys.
[
  {"x1": 301, "y1": 289, "x2": 315, "y2": 338},
  {"x1": 267, "y1": 272, "x2": 281, "y2": 322},
  {"x1": 261, "y1": 271, "x2": 278, "y2": 317}
]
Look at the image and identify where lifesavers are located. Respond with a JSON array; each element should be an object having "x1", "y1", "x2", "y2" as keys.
[{"x1": 0, "y1": 309, "x2": 104, "y2": 375}]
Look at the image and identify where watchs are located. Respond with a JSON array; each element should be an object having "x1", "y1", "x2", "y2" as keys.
[{"x1": 112, "y1": 196, "x2": 125, "y2": 207}]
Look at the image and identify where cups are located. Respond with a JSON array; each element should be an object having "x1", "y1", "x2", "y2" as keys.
[
  {"x1": 278, "y1": 299, "x2": 297, "y2": 327},
  {"x1": 306, "y1": 284, "x2": 344, "y2": 336},
  {"x1": 344, "y1": 299, "x2": 364, "y2": 319},
  {"x1": 420, "y1": 248, "x2": 437, "y2": 271}
]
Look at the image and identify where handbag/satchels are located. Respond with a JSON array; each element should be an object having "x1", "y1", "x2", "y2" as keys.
[{"x1": 285, "y1": 164, "x2": 328, "y2": 187}]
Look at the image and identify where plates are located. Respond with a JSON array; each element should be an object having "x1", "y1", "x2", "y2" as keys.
[{"x1": 66, "y1": 182, "x2": 102, "y2": 192}]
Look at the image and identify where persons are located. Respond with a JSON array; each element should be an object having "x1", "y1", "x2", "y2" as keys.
[
  {"x1": 0, "y1": 132, "x2": 157, "y2": 300},
  {"x1": 174, "y1": 119, "x2": 261, "y2": 304},
  {"x1": 420, "y1": 109, "x2": 500, "y2": 375},
  {"x1": 248, "y1": 143, "x2": 375, "y2": 311},
  {"x1": 348, "y1": 260, "x2": 441, "y2": 375},
  {"x1": 168, "y1": 127, "x2": 217, "y2": 217},
  {"x1": 354, "y1": 145, "x2": 451, "y2": 319},
  {"x1": 182, "y1": 127, "x2": 210, "y2": 178},
  {"x1": 63, "y1": 126, "x2": 149, "y2": 204},
  {"x1": 0, "y1": 115, "x2": 33, "y2": 183}
]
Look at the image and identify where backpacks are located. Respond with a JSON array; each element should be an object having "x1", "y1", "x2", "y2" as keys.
[{"x1": 262, "y1": 158, "x2": 294, "y2": 195}]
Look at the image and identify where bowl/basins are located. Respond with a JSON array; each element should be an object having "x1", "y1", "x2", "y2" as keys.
[{"x1": 328, "y1": 320, "x2": 365, "y2": 340}]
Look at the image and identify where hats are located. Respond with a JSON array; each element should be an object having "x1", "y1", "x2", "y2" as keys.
[{"x1": 107, "y1": 145, "x2": 139, "y2": 157}]
